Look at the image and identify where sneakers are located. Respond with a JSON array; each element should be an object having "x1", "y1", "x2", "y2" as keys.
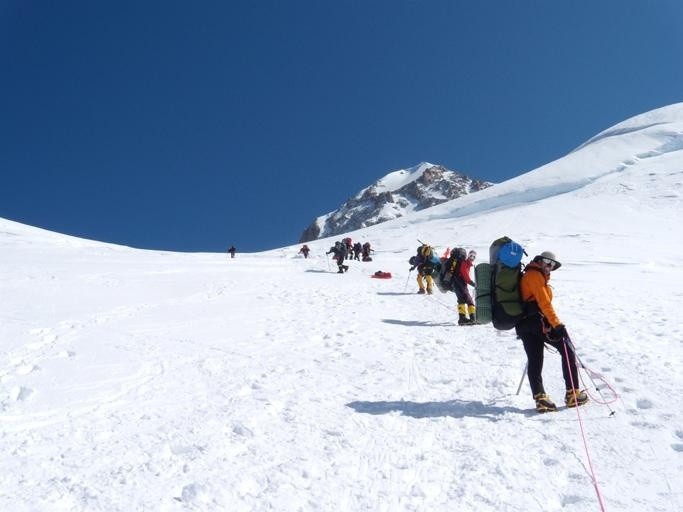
[
  {"x1": 564, "y1": 390, "x2": 588, "y2": 407},
  {"x1": 345, "y1": 257, "x2": 360, "y2": 261},
  {"x1": 427, "y1": 289, "x2": 432, "y2": 294},
  {"x1": 418, "y1": 289, "x2": 425, "y2": 294},
  {"x1": 535, "y1": 394, "x2": 557, "y2": 413}
]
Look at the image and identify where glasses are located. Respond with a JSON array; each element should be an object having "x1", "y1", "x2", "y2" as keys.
[
  {"x1": 468, "y1": 254, "x2": 476, "y2": 258},
  {"x1": 542, "y1": 258, "x2": 556, "y2": 266}
]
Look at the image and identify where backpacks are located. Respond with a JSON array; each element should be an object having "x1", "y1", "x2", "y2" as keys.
[
  {"x1": 442, "y1": 254, "x2": 459, "y2": 287},
  {"x1": 488, "y1": 236, "x2": 526, "y2": 330}
]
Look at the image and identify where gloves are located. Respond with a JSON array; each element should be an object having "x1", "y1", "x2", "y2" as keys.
[{"x1": 555, "y1": 327, "x2": 568, "y2": 339}]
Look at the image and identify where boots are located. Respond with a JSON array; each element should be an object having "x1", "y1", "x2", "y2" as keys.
[
  {"x1": 458, "y1": 314, "x2": 472, "y2": 324},
  {"x1": 337, "y1": 267, "x2": 343, "y2": 273},
  {"x1": 471, "y1": 314, "x2": 479, "y2": 322},
  {"x1": 342, "y1": 265, "x2": 349, "y2": 272}
]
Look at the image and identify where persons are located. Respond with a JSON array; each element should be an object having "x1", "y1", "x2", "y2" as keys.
[
  {"x1": 326, "y1": 237, "x2": 374, "y2": 274},
  {"x1": 453, "y1": 250, "x2": 481, "y2": 326},
  {"x1": 409, "y1": 244, "x2": 434, "y2": 295},
  {"x1": 228, "y1": 246, "x2": 236, "y2": 258},
  {"x1": 297, "y1": 245, "x2": 311, "y2": 259},
  {"x1": 515, "y1": 251, "x2": 588, "y2": 412}
]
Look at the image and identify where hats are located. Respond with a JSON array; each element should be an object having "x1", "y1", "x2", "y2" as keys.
[{"x1": 534, "y1": 250, "x2": 561, "y2": 270}]
